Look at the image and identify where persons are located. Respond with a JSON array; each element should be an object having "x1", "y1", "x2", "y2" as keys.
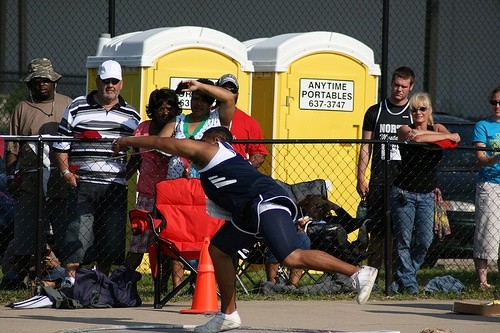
[
  {"x1": 208, "y1": 73, "x2": 269, "y2": 171},
  {"x1": 51, "y1": 60, "x2": 143, "y2": 288},
  {"x1": 261, "y1": 193, "x2": 355, "y2": 296},
  {"x1": 158, "y1": 78, "x2": 236, "y2": 296},
  {"x1": 5, "y1": 58, "x2": 74, "y2": 290},
  {"x1": 385, "y1": 91, "x2": 461, "y2": 298},
  {"x1": 111, "y1": 126, "x2": 378, "y2": 333},
  {"x1": 356, "y1": 66, "x2": 452, "y2": 290},
  {"x1": 470, "y1": 87, "x2": 500, "y2": 290},
  {"x1": 112, "y1": 87, "x2": 183, "y2": 297}
]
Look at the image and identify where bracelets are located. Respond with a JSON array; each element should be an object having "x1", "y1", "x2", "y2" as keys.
[{"x1": 59, "y1": 169, "x2": 69, "y2": 177}]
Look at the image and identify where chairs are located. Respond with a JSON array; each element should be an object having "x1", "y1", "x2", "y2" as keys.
[
  {"x1": 150, "y1": 178, "x2": 239, "y2": 315},
  {"x1": 238, "y1": 177, "x2": 330, "y2": 295}
]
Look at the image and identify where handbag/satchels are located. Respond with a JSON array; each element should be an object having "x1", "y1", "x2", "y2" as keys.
[
  {"x1": 73, "y1": 267, "x2": 143, "y2": 308},
  {"x1": 16, "y1": 168, "x2": 50, "y2": 198}
]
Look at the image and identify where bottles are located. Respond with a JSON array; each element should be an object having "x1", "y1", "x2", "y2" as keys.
[{"x1": 357, "y1": 196, "x2": 367, "y2": 219}]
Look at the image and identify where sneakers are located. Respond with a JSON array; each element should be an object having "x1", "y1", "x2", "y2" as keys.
[
  {"x1": 194, "y1": 311, "x2": 241, "y2": 333},
  {"x1": 392, "y1": 281, "x2": 418, "y2": 295},
  {"x1": 350, "y1": 264, "x2": 378, "y2": 305}
]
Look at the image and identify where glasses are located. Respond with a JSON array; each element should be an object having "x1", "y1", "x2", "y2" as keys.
[
  {"x1": 226, "y1": 88, "x2": 238, "y2": 94},
  {"x1": 32, "y1": 79, "x2": 50, "y2": 83},
  {"x1": 100, "y1": 78, "x2": 119, "y2": 85},
  {"x1": 410, "y1": 107, "x2": 429, "y2": 111},
  {"x1": 489, "y1": 100, "x2": 500, "y2": 105}
]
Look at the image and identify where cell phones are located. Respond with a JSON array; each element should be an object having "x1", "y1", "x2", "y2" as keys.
[{"x1": 175, "y1": 83, "x2": 189, "y2": 94}]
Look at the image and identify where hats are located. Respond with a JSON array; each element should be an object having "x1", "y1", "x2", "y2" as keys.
[
  {"x1": 96, "y1": 60, "x2": 123, "y2": 80},
  {"x1": 218, "y1": 74, "x2": 239, "y2": 88},
  {"x1": 19, "y1": 58, "x2": 62, "y2": 82}
]
